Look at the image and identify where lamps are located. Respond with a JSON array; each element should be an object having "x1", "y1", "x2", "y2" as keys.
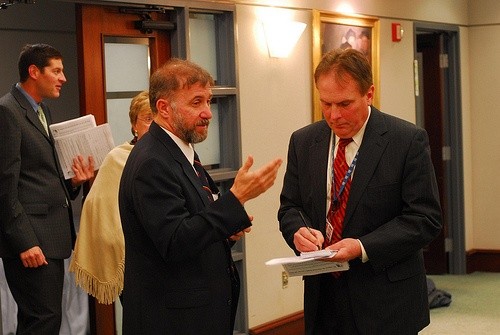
[{"x1": 262, "y1": 16, "x2": 307, "y2": 58}]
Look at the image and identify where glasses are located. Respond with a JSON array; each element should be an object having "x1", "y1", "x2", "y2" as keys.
[{"x1": 137, "y1": 117, "x2": 153, "y2": 124}]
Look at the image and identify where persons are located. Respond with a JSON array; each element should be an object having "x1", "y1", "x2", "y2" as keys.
[
  {"x1": 67, "y1": 90, "x2": 154, "y2": 308},
  {"x1": 0, "y1": 43, "x2": 95, "y2": 335},
  {"x1": 277, "y1": 48, "x2": 445, "y2": 335},
  {"x1": 118, "y1": 58, "x2": 284, "y2": 335}
]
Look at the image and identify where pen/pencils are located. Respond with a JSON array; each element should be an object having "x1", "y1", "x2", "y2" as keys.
[{"x1": 298, "y1": 210, "x2": 321, "y2": 250}]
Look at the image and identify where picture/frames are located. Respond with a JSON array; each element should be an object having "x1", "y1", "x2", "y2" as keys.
[{"x1": 311, "y1": 8, "x2": 381, "y2": 122}]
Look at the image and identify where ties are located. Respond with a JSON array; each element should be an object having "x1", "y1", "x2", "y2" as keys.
[
  {"x1": 193, "y1": 151, "x2": 214, "y2": 205},
  {"x1": 324, "y1": 138, "x2": 354, "y2": 278},
  {"x1": 37, "y1": 105, "x2": 49, "y2": 136}
]
